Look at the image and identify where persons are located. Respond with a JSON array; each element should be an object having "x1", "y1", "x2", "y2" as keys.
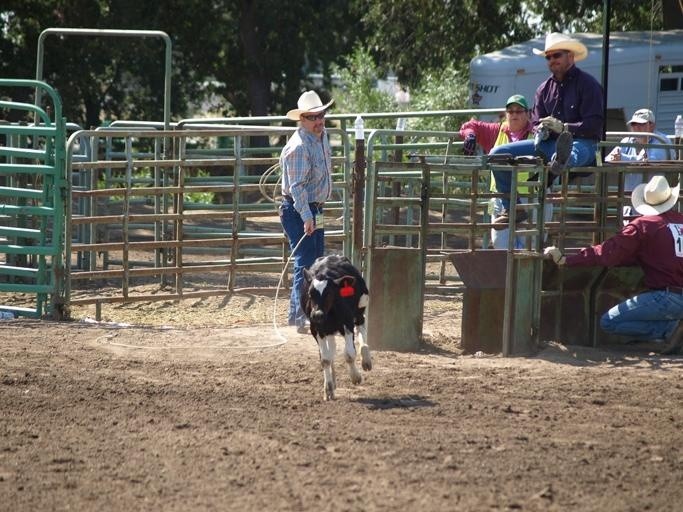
[
  {"x1": 460, "y1": 93, "x2": 553, "y2": 252},
  {"x1": 278, "y1": 89, "x2": 335, "y2": 334},
  {"x1": 544, "y1": 176, "x2": 683, "y2": 355},
  {"x1": 488, "y1": 32, "x2": 606, "y2": 230},
  {"x1": 604, "y1": 108, "x2": 677, "y2": 230}
]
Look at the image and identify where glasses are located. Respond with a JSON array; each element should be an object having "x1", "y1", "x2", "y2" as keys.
[
  {"x1": 545, "y1": 53, "x2": 561, "y2": 60},
  {"x1": 508, "y1": 111, "x2": 523, "y2": 114},
  {"x1": 301, "y1": 112, "x2": 325, "y2": 120}
]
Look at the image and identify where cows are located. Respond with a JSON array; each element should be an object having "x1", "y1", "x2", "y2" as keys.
[{"x1": 300, "y1": 254, "x2": 372, "y2": 401}]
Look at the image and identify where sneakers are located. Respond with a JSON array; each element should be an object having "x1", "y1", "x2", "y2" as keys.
[
  {"x1": 493, "y1": 203, "x2": 528, "y2": 229},
  {"x1": 550, "y1": 132, "x2": 573, "y2": 175},
  {"x1": 661, "y1": 321, "x2": 682, "y2": 355}
]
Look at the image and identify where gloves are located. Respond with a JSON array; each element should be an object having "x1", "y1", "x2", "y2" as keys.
[
  {"x1": 536, "y1": 116, "x2": 568, "y2": 140},
  {"x1": 462, "y1": 133, "x2": 476, "y2": 156},
  {"x1": 545, "y1": 245, "x2": 566, "y2": 266}
]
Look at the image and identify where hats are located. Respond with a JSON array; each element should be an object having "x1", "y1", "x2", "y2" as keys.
[
  {"x1": 506, "y1": 95, "x2": 528, "y2": 109},
  {"x1": 287, "y1": 91, "x2": 334, "y2": 120},
  {"x1": 533, "y1": 32, "x2": 587, "y2": 61},
  {"x1": 631, "y1": 176, "x2": 680, "y2": 216},
  {"x1": 627, "y1": 109, "x2": 655, "y2": 124}
]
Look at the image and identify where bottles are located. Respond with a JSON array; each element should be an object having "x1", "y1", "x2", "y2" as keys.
[
  {"x1": 354, "y1": 115, "x2": 364, "y2": 139},
  {"x1": 674, "y1": 116, "x2": 682, "y2": 137}
]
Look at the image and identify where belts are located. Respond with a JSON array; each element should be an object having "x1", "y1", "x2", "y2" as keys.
[{"x1": 283, "y1": 196, "x2": 324, "y2": 209}]
